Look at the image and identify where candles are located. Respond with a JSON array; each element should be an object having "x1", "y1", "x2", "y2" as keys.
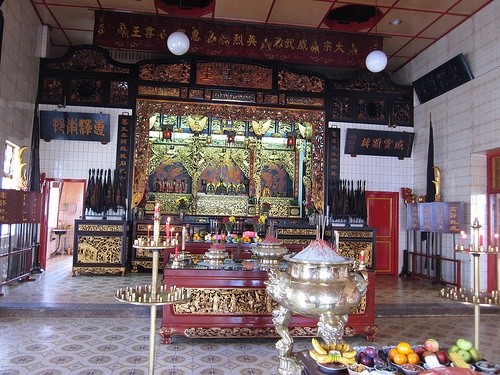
[
  {"x1": 460, "y1": 230, "x2": 483, "y2": 247},
  {"x1": 361, "y1": 251, "x2": 364, "y2": 260},
  {"x1": 146, "y1": 206, "x2": 186, "y2": 258},
  {"x1": 335, "y1": 232, "x2": 340, "y2": 246}
]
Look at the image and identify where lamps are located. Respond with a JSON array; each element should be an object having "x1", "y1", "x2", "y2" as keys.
[
  {"x1": 287, "y1": 132, "x2": 297, "y2": 148},
  {"x1": 162, "y1": 124, "x2": 174, "y2": 141},
  {"x1": 227, "y1": 131, "x2": 236, "y2": 142},
  {"x1": 365, "y1": 0, "x2": 387, "y2": 72},
  {"x1": 167, "y1": 0, "x2": 190, "y2": 55}
]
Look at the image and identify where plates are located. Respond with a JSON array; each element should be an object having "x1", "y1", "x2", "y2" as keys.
[
  {"x1": 360, "y1": 363, "x2": 375, "y2": 369},
  {"x1": 316, "y1": 361, "x2": 347, "y2": 370},
  {"x1": 387, "y1": 357, "x2": 402, "y2": 367},
  {"x1": 419, "y1": 360, "x2": 449, "y2": 365}
]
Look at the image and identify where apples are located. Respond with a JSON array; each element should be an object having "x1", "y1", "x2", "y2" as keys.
[
  {"x1": 447, "y1": 338, "x2": 481, "y2": 362},
  {"x1": 232, "y1": 236, "x2": 240, "y2": 242},
  {"x1": 413, "y1": 339, "x2": 448, "y2": 364}
]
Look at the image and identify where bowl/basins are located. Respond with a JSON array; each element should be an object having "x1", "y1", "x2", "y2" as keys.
[
  {"x1": 450, "y1": 362, "x2": 475, "y2": 371},
  {"x1": 374, "y1": 364, "x2": 398, "y2": 374},
  {"x1": 423, "y1": 363, "x2": 445, "y2": 370},
  {"x1": 346, "y1": 364, "x2": 371, "y2": 375},
  {"x1": 401, "y1": 364, "x2": 425, "y2": 375},
  {"x1": 475, "y1": 361, "x2": 500, "y2": 373}
]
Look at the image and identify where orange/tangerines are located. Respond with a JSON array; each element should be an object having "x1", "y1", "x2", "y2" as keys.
[
  {"x1": 244, "y1": 236, "x2": 251, "y2": 242},
  {"x1": 388, "y1": 342, "x2": 419, "y2": 364},
  {"x1": 193, "y1": 233, "x2": 201, "y2": 240}
]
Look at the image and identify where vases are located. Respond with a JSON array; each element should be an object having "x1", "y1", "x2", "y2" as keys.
[
  {"x1": 253, "y1": 232, "x2": 258, "y2": 242},
  {"x1": 179, "y1": 208, "x2": 184, "y2": 219},
  {"x1": 263, "y1": 212, "x2": 267, "y2": 220},
  {"x1": 225, "y1": 231, "x2": 231, "y2": 243}
]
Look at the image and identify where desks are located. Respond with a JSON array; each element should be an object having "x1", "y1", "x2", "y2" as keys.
[{"x1": 160, "y1": 262, "x2": 377, "y2": 341}]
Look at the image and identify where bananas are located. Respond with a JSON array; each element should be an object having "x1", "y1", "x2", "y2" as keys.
[{"x1": 308, "y1": 337, "x2": 356, "y2": 365}]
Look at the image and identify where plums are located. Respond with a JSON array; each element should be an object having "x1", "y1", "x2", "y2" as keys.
[{"x1": 358, "y1": 347, "x2": 383, "y2": 365}]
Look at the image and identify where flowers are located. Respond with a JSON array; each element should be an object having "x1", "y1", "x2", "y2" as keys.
[
  {"x1": 261, "y1": 201, "x2": 272, "y2": 212},
  {"x1": 253, "y1": 215, "x2": 265, "y2": 232},
  {"x1": 222, "y1": 215, "x2": 235, "y2": 231},
  {"x1": 176, "y1": 196, "x2": 190, "y2": 208}
]
